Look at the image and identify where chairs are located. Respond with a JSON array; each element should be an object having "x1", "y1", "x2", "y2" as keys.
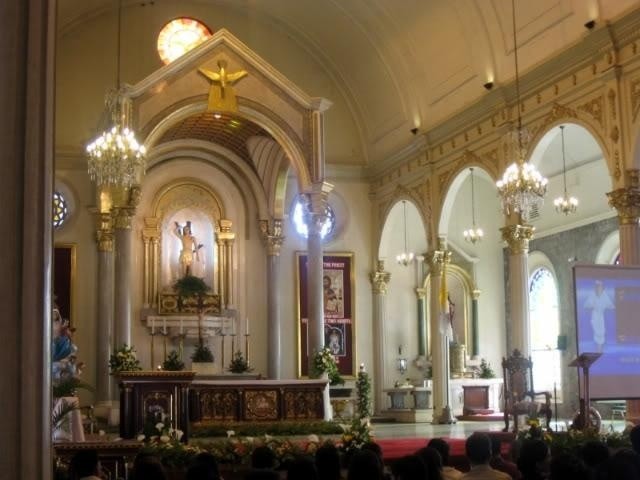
[{"x1": 502, "y1": 348, "x2": 554, "y2": 432}]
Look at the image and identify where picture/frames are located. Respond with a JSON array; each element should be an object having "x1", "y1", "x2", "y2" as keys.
[
  {"x1": 53, "y1": 241, "x2": 76, "y2": 344},
  {"x1": 294, "y1": 250, "x2": 357, "y2": 380}
]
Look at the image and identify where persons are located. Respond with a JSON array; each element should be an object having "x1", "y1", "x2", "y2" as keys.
[
  {"x1": 582, "y1": 277, "x2": 616, "y2": 355},
  {"x1": 328, "y1": 326, "x2": 341, "y2": 355},
  {"x1": 173, "y1": 224, "x2": 200, "y2": 279},
  {"x1": 324, "y1": 275, "x2": 339, "y2": 318}
]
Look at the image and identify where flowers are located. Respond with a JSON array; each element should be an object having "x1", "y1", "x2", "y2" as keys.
[
  {"x1": 309, "y1": 346, "x2": 337, "y2": 376},
  {"x1": 97, "y1": 415, "x2": 376, "y2": 471},
  {"x1": 107, "y1": 342, "x2": 143, "y2": 374}
]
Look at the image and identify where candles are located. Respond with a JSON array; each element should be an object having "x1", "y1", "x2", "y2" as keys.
[{"x1": 151, "y1": 315, "x2": 249, "y2": 335}]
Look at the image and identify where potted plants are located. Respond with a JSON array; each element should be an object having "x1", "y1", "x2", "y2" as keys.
[{"x1": 190, "y1": 337, "x2": 217, "y2": 374}]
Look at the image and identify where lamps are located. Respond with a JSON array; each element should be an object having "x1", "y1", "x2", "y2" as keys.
[
  {"x1": 553, "y1": 126, "x2": 578, "y2": 216},
  {"x1": 396, "y1": 201, "x2": 418, "y2": 267},
  {"x1": 496, "y1": 1, "x2": 548, "y2": 256},
  {"x1": 432, "y1": 235, "x2": 451, "y2": 266},
  {"x1": 463, "y1": 167, "x2": 484, "y2": 246},
  {"x1": 86, "y1": 0, "x2": 148, "y2": 189}
]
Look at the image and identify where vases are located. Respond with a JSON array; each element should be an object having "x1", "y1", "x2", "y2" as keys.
[{"x1": 314, "y1": 370, "x2": 328, "y2": 379}]
